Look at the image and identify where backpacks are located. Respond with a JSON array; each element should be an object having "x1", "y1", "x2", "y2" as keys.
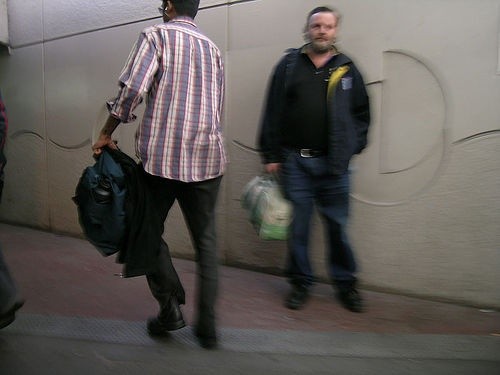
[{"x1": 73, "y1": 146, "x2": 143, "y2": 257}]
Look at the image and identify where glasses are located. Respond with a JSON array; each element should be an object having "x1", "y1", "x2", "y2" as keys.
[{"x1": 158, "y1": 3, "x2": 170, "y2": 13}]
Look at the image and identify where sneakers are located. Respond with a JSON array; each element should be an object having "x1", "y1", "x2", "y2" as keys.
[
  {"x1": 285, "y1": 282, "x2": 309, "y2": 308},
  {"x1": 339, "y1": 287, "x2": 364, "y2": 313}
]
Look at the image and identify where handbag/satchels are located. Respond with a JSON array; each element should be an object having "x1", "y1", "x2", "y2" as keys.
[{"x1": 241, "y1": 166, "x2": 295, "y2": 241}]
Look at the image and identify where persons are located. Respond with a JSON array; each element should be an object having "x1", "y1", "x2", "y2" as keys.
[
  {"x1": 0, "y1": 95, "x2": 27, "y2": 330},
  {"x1": 257, "y1": 7, "x2": 371, "y2": 312},
  {"x1": 91, "y1": 0, "x2": 227, "y2": 350}
]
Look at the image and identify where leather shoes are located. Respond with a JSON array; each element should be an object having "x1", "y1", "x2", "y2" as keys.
[
  {"x1": 148, "y1": 308, "x2": 186, "y2": 333},
  {"x1": 193, "y1": 314, "x2": 217, "y2": 348}
]
